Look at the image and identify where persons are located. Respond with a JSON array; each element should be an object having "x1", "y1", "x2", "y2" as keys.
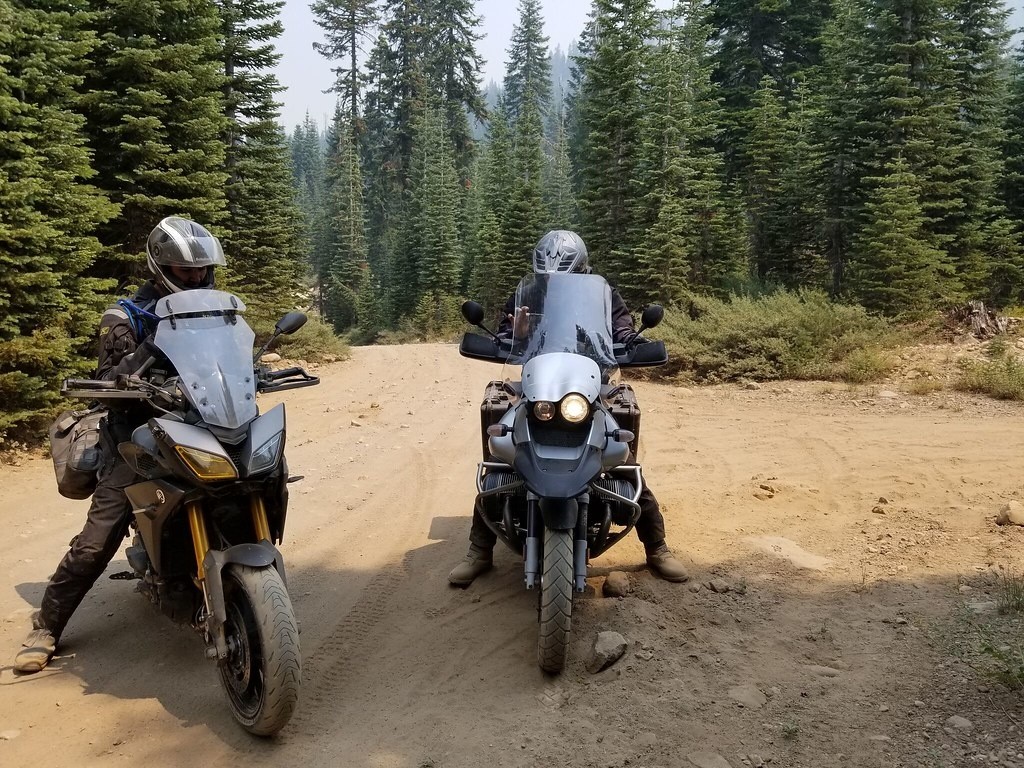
[
  {"x1": 449, "y1": 229, "x2": 688, "y2": 584},
  {"x1": 14, "y1": 217, "x2": 227, "y2": 671}
]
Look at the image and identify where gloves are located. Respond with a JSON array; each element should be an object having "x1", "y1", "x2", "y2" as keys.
[{"x1": 624, "y1": 332, "x2": 652, "y2": 356}]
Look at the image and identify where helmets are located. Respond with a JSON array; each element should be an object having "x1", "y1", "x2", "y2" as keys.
[
  {"x1": 532, "y1": 230, "x2": 589, "y2": 274},
  {"x1": 145, "y1": 216, "x2": 227, "y2": 294}
]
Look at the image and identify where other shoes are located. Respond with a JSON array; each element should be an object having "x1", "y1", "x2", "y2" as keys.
[
  {"x1": 13, "y1": 628, "x2": 57, "y2": 672},
  {"x1": 447, "y1": 549, "x2": 493, "y2": 585},
  {"x1": 645, "y1": 543, "x2": 689, "y2": 582}
]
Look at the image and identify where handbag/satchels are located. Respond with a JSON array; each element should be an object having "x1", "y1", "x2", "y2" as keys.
[{"x1": 47, "y1": 409, "x2": 109, "y2": 500}]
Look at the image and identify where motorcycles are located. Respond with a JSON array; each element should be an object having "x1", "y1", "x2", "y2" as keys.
[
  {"x1": 60, "y1": 290, "x2": 320, "y2": 737},
  {"x1": 460, "y1": 274, "x2": 668, "y2": 673}
]
